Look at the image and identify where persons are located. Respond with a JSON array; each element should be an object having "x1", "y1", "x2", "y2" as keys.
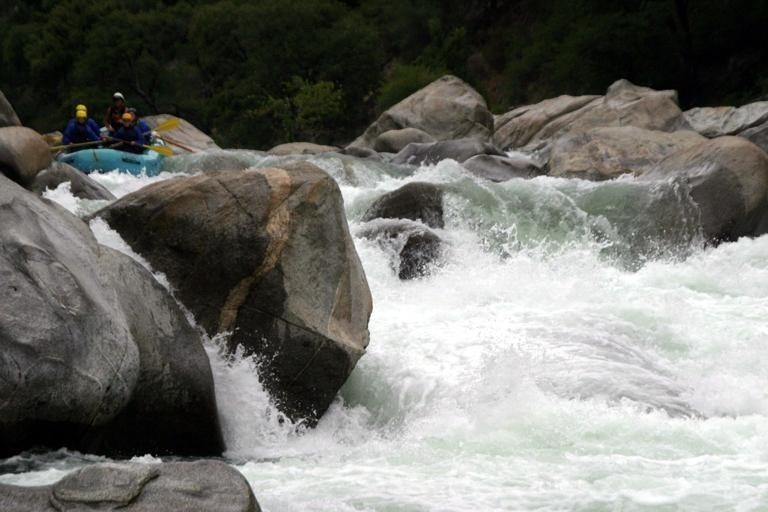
[{"x1": 62, "y1": 93, "x2": 152, "y2": 155}]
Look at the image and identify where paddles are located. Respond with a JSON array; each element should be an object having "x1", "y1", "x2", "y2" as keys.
[
  {"x1": 111, "y1": 137, "x2": 173, "y2": 157},
  {"x1": 109, "y1": 117, "x2": 180, "y2": 146}
]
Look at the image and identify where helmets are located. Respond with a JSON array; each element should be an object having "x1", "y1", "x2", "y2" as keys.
[
  {"x1": 112, "y1": 92, "x2": 124, "y2": 103},
  {"x1": 76, "y1": 104, "x2": 87, "y2": 111},
  {"x1": 122, "y1": 113, "x2": 133, "y2": 122},
  {"x1": 76, "y1": 110, "x2": 87, "y2": 118},
  {"x1": 128, "y1": 108, "x2": 138, "y2": 116}
]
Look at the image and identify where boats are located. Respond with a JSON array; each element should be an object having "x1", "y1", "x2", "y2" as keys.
[{"x1": 54, "y1": 125, "x2": 165, "y2": 178}]
